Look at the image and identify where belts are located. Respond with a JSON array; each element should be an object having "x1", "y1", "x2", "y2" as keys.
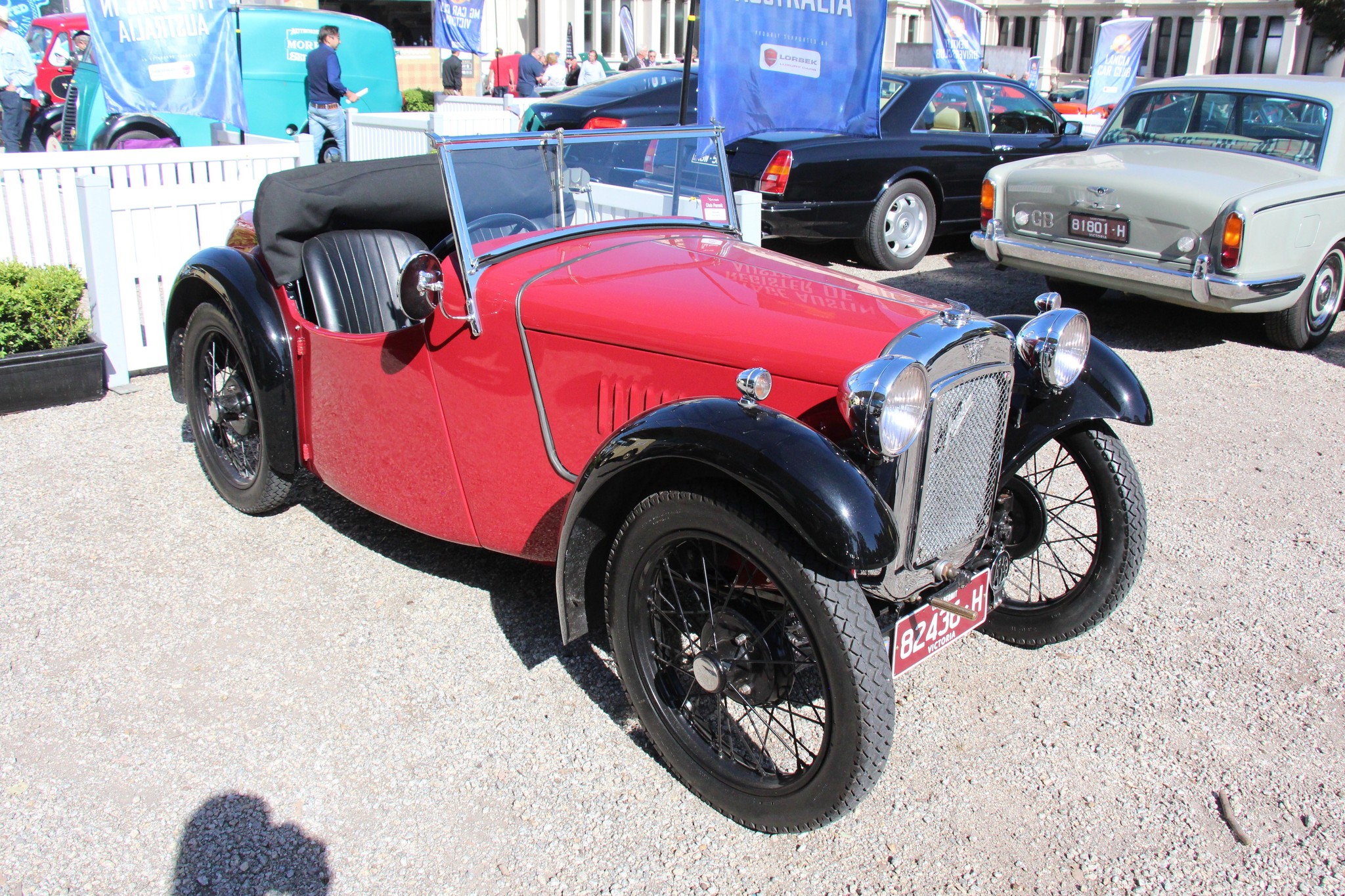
[{"x1": 310, "y1": 102, "x2": 340, "y2": 109}]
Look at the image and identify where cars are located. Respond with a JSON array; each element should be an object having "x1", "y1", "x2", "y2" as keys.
[
  {"x1": 1046, "y1": 85, "x2": 1090, "y2": 104},
  {"x1": 968, "y1": 73, "x2": 1345, "y2": 350},
  {"x1": 19, "y1": 3, "x2": 403, "y2": 158},
  {"x1": 166, "y1": 111, "x2": 1159, "y2": 836},
  {"x1": 628, "y1": 65, "x2": 1098, "y2": 274},
  {"x1": 518, "y1": 62, "x2": 700, "y2": 175}
]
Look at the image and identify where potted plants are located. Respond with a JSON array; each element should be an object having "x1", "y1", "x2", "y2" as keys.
[{"x1": 0, "y1": 256, "x2": 108, "y2": 416}]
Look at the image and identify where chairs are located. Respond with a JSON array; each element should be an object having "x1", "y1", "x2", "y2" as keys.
[
  {"x1": 299, "y1": 224, "x2": 431, "y2": 336},
  {"x1": 929, "y1": 104, "x2": 974, "y2": 134},
  {"x1": 470, "y1": 215, "x2": 554, "y2": 246}
]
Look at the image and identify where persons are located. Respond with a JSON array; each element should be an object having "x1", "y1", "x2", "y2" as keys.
[
  {"x1": 0, "y1": 5, "x2": 96, "y2": 153},
  {"x1": 485, "y1": 48, "x2": 514, "y2": 98},
  {"x1": 680, "y1": 44, "x2": 697, "y2": 63},
  {"x1": 306, "y1": 26, "x2": 360, "y2": 164},
  {"x1": 517, "y1": 46, "x2": 606, "y2": 98},
  {"x1": 1010, "y1": 71, "x2": 1089, "y2": 95},
  {"x1": 442, "y1": 50, "x2": 463, "y2": 96},
  {"x1": 618, "y1": 45, "x2": 659, "y2": 71},
  {"x1": 980, "y1": 62, "x2": 996, "y2": 111}
]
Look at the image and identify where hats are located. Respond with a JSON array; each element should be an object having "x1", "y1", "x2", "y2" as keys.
[{"x1": 0, "y1": 5, "x2": 18, "y2": 28}]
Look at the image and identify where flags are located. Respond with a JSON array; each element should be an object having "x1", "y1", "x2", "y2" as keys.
[{"x1": 618, "y1": 5, "x2": 636, "y2": 61}]
